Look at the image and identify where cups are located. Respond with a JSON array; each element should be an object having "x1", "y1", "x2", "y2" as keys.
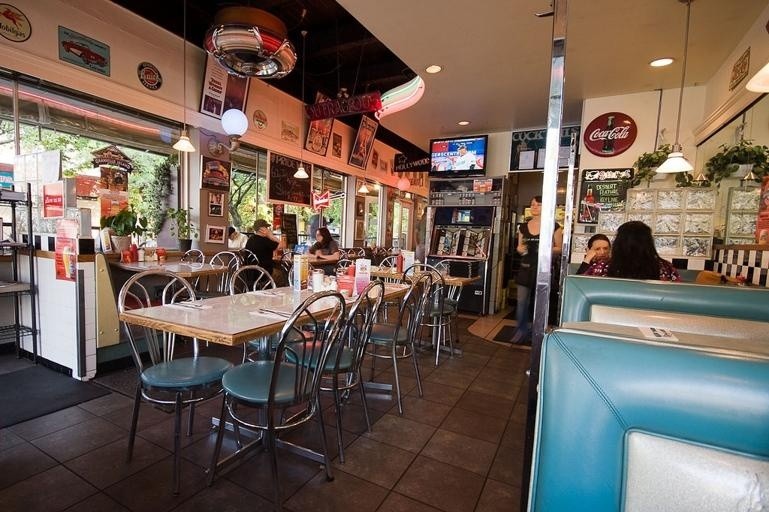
[{"x1": 336, "y1": 275, "x2": 355, "y2": 298}]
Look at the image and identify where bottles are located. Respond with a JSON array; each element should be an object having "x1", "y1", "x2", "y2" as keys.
[
  {"x1": 137, "y1": 248, "x2": 145, "y2": 261},
  {"x1": 348, "y1": 259, "x2": 356, "y2": 276},
  {"x1": 414, "y1": 258, "x2": 421, "y2": 273},
  {"x1": 130, "y1": 244, "x2": 137, "y2": 261},
  {"x1": 123, "y1": 249, "x2": 132, "y2": 264},
  {"x1": 157, "y1": 247, "x2": 167, "y2": 260},
  {"x1": 312, "y1": 269, "x2": 325, "y2": 293},
  {"x1": 62, "y1": 246, "x2": 72, "y2": 280},
  {"x1": 397, "y1": 251, "x2": 402, "y2": 274}
]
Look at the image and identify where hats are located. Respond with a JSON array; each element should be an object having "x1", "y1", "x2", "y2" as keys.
[{"x1": 254, "y1": 219, "x2": 271, "y2": 231}]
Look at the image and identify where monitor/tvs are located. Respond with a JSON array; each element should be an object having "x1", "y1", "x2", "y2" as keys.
[{"x1": 428, "y1": 134, "x2": 487, "y2": 176}]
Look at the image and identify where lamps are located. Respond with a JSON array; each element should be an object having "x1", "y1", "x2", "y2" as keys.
[
  {"x1": 207, "y1": 102, "x2": 248, "y2": 156},
  {"x1": 393, "y1": 170, "x2": 413, "y2": 193},
  {"x1": 358, "y1": 82, "x2": 370, "y2": 193},
  {"x1": 292, "y1": 28, "x2": 310, "y2": 180},
  {"x1": 172, "y1": 0, "x2": 202, "y2": 154},
  {"x1": 657, "y1": 1, "x2": 697, "y2": 174}
]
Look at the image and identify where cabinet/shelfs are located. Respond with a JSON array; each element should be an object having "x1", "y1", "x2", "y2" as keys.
[
  {"x1": 0, "y1": 181, "x2": 41, "y2": 364},
  {"x1": 421, "y1": 178, "x2": 507, "y2": 317}
]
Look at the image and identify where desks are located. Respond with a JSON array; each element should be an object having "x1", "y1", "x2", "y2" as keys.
[{"x1": 119, "y1": 281, "x2": 412, "y2": 477}]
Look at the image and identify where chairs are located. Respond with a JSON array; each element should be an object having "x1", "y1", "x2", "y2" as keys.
[
  {"x1": 102, "y1": 239, "x2": 481, "y2": 414},
  {"x1": 205, "y1": 290, "x2": 346, "y2": 512},
  {"x1": 284, "y1": 281, "x2": 384, "y2": 463},
  {"x1": 117, "y1": 269, "x2": 241, "y2": 494}
]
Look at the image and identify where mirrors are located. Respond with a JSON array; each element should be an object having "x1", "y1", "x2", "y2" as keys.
[{"x1": 696, "y1": 93, "x2": 769, "y2": 268}]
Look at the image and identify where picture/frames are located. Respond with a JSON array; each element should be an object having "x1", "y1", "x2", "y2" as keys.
[
  {"x1": 198, "y1": 50, "x2": 253, "y2": 120},
  {"x1": 198, "y1": 154, "x2": 232, "y2": 192},
  {"x1": 354, "y1": 179, "x2": 366, "y2": 242},
  {"x1": 347, "y1": 114, "x2": 379, "y2": 169},
  {"x1": 204, "y1": 225, "x2": 228, "y2": 245},
  {"x1": 207, "y1": 191, "x2": 224, "y2": 218},
  {"x1": 304, "y1": 86, "x2": 337, "y2": 157}
]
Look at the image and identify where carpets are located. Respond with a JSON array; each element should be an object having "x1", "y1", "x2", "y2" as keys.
[
  {"x1": 91, "y1": 346, "x2": 259, "y2": 415},
  {"x1": 1, "y1": 362, "x2": 112, "y2": 429}
]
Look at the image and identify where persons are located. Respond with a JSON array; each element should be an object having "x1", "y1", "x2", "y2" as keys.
[
  {"x1": 244, "y1": 219, "x2": 285, "y2": 289},
  {"x1": 304, "y1": 228, "x2": 340, "y2": 276},
  {"x1": 510, "y1": 195, "x2": 563, "y2": 344},
  {"x1": 575, "y1": 234, "x2": 611, "y2": 275},
  {"x1": 228, "y1": 227, "x2": 249, "y2": 250},
  {"x1": 580, "y1": 221, "x2": 683, "y2": 283},
  {"x1": 446, "y1": 146, "x2": 475, "y2": 171}
]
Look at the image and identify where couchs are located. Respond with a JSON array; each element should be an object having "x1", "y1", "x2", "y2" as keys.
[{"x1": 525, "y1": 255, "x2": 766, "y2": 512}]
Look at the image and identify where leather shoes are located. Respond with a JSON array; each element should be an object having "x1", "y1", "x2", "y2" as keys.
[{"x1": 508, "y1": 329, "x2": 527, "y2": 344}]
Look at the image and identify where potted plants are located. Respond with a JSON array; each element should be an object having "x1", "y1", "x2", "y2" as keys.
[
  {"x1": 627, "y1": 137, "x2": 711, "y2": 188},
  {"x1": 701, "y1": 138, "x2": 769, "y2": 188},
  {"x1": 165, "y1": 202, "x2": 202, "y2": 253},
  {"x1": 106, "y1": 200, "x2": 144, "y2": 251}
]
